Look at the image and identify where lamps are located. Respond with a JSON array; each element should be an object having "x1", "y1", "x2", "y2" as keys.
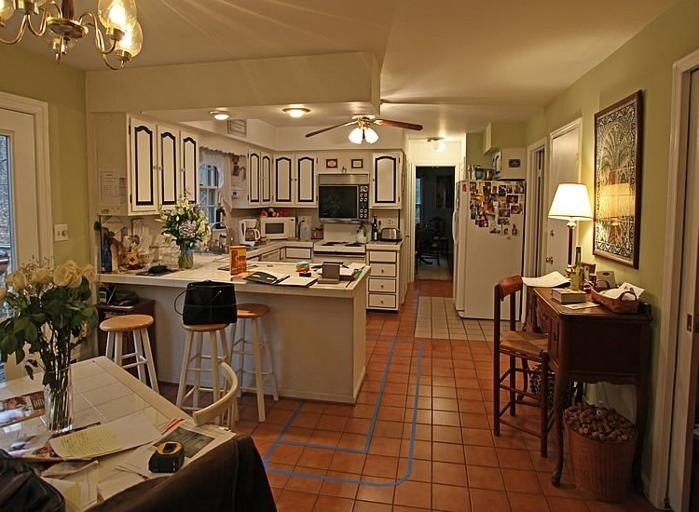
[
  {"x1": 348, "y1": 118, "x2": 379, "y2": 144},
  {"x1": 210, "y1": 111, "x2": 229, "y2": 120},
  {"x1": 1, "y1": 0, "x2": 145, "y2": 71},
  {"x1": 547, "y1": 183, "x2": 593, "y2": 271},
  {"x1": 281, "y1": 107, "x2": 311, "y2": 117},
  {"x1": 427, "y1": 137, "x2": 445, "y2": 150}
]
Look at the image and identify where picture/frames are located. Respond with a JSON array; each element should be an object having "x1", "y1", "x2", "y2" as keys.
[{"x1": 592, "y1": 90, "x2": 644, "y2": 270}]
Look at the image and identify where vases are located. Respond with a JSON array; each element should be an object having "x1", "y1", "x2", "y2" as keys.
[
  {"x1": 41, "y1": 360, "x2": 75, "y2": 435},
  {"x1": 177, "y1": 242, "x2": 194, "y2": 269}
]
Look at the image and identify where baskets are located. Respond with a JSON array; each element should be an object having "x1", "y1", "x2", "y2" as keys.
[{"x1": 563, "y1": 404, "x2": 637, "y2": 503}]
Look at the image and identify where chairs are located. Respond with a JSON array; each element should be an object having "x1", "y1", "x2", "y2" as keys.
[
  {"x1": 429, "y1": 216, "x2": 449, "y2": 265},
  {"x1": 493, "y1": 275, "x2": 571, "y2": 458}
]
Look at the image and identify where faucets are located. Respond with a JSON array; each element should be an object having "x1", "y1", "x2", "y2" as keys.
[{"x1": 210, "y1": 222, "x2": 229, "y2": 242}]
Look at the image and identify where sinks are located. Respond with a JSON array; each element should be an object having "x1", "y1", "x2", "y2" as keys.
[{"x1": 213, "y1": 246, "x2": 257, "y2": 255}]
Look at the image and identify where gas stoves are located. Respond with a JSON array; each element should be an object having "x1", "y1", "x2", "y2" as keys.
[{"x1": 314, "y1": 239, "x2": 371, "y2": 255}]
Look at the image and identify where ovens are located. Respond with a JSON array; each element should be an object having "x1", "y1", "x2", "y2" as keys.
[{"x1": 318, "y1": 174, "x2": 371, "y2": 225}]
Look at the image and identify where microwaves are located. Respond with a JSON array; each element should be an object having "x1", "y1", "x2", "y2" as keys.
[{"x1": 260, "y1": 216, "x2": 296, "y2": 240}]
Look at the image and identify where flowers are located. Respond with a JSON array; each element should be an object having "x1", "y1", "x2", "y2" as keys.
[
  {"x1": 155, "y1": 188, "x2": 212, "y2": 247},
  {"x1": 0, "y1": 255, "x2": 99, "y2": 430}
]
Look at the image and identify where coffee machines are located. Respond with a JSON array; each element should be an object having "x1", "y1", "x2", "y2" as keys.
[{"x1": 238, "y1": 219, "x2": 260, "y2": 247}]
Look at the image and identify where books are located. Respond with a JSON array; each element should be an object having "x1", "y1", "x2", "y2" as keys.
[
  {"x1": 521, "y1": 271, "x2": 571, "y2": 288},
  {"x1": 243, "y1": 268, "x2": 316, "y2": 288}
]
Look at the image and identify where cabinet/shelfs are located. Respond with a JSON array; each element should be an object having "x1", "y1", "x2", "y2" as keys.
[
  {"x1": 367, "y1": 249, "x2": 400, "y2": 315},
  {"x1": 369, "y1": 151, "x2": 402, "y2": 210},
  {"x1": 247, "y1": 151, "x2": 274, "y2": 207},
  {"x1": 275, "y1": 148, "x2": 318, "y2": 208},
  {"x1": 97, "y1": 239, "x2": 371, "y2": 404},
  {"x1": 88, "y1": 124, "x2": 198, "y2": 215}
]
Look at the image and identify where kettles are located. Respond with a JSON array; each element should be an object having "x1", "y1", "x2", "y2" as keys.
[{"x1": 356, "y1": 225, "x2": 370, "y2": 244}]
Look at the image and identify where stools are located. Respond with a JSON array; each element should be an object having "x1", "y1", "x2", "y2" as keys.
[
  {"x1": 229, "y1": 304, "x2": 280, "y2": 422},
  {"x1": 99, "y1": 315, "x2": 159, "y2": 394},
  {"x1": 175, "y1": 324, "x2": 240, "y2": 430}
]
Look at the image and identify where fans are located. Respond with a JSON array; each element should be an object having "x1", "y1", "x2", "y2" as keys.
[{"x1": 305, "y1": 115, "x2": 423, "y2": 144}]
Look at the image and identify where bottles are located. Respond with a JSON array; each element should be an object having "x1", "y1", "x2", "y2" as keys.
[
  {"x1": 229, "y1": 246, "x2": 247, "y2": 275},
  {"x1": 216, "y1": 202, "x2": 229, "y2": 229},
  {"x1": 372, "y1": 216, "x2": 379, "y2": 240},
  {"x1": 232, "y1": 192, "x2": 238, "y2": 206},
  {"x1": 571, "y1": 246, "x2": 584, "y2": 291}
]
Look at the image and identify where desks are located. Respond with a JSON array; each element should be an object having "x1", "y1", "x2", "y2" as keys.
[
  {"x1": 0, "y1": 357, "x2": 252, "y2": 512},
  {"x1": 525, "y1": 288, "x2": 653, "y2": 490}
]
